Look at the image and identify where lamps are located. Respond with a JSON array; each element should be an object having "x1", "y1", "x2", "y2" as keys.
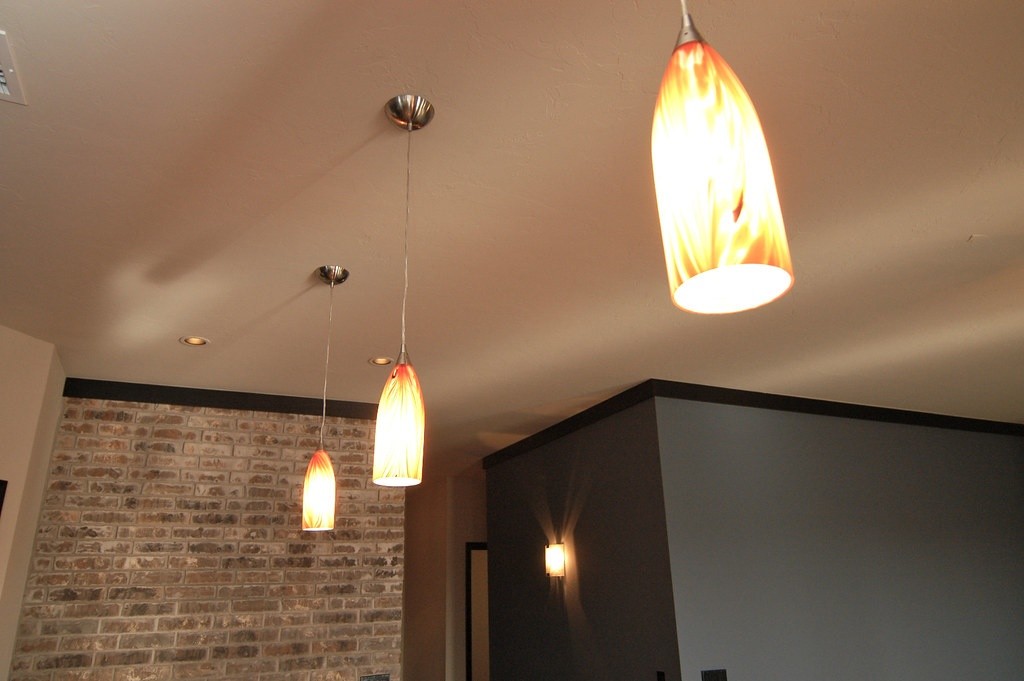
[
  {"x1": 299, "y1": 265, "x2": 350, "y2": 534},
  {"x1": 371, "y1": 95, "x2": 434, "y2": 489},
  {"x1": 651, "y1": 0, "x2": 796, "y2": 317}
]
